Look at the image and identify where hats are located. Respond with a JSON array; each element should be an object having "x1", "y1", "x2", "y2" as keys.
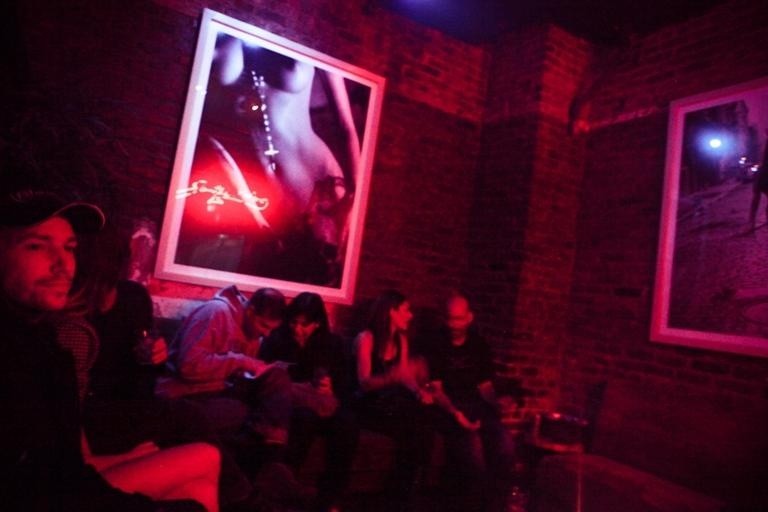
[{"x1": 0, "y1": 182, "x2": 105, "y2": 238}]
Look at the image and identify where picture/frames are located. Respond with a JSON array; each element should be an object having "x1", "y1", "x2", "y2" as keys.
[
  {"x1": 647, "y1": 73, "x2": 767, "y2": 362},
  {"x1": 152, "y1": 5, "x2": 389, "y2": 306}
]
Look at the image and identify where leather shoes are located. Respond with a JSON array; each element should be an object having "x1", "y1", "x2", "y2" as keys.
[{"x1": 254, "y1": 463, "x2": 317, "y2": 504}]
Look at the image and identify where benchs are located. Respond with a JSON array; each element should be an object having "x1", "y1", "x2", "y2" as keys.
[{"x1": 532, "y1": 377, "x2": 768, "y2": 510}]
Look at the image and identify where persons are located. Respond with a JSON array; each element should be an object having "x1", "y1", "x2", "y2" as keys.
[
  {"x1": 155, "y1": 288, "x2": 577, "y2": 511},
  {"x1": 211, "y1": 23, "x2": 359, "y2": 211},
  {"x1": 737, "y1": 142, "x2": 768, "y2": 234},
  {"x1": 1, "y1": 180, "x2": 224, "y2": 512}
]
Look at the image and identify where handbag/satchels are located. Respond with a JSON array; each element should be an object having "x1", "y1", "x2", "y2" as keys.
[{"x1": 236, "y1": 363, "x2": 291, "y2": 418}]
